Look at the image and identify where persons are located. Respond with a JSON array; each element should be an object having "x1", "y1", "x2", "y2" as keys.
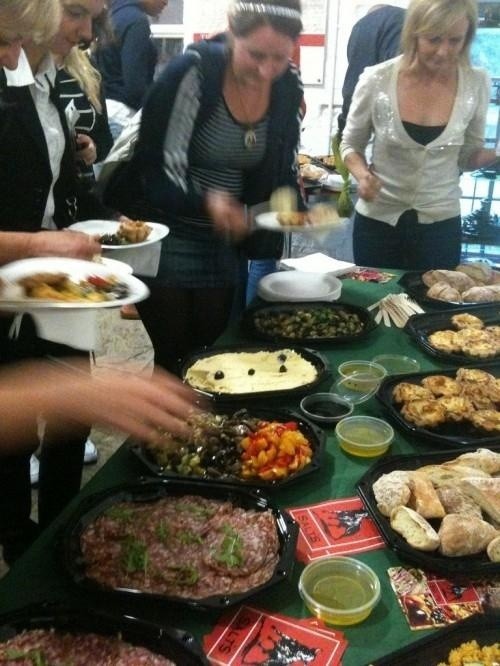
[
  {"x1": 1, "y1": 0, "x2": 311, "y2": 563},
  {"x1": 338, "y1": 0, "x2": 500, "y2": 269},
  {"x1": 337, "y1": 4, "x2": 407, "y2": 139}
]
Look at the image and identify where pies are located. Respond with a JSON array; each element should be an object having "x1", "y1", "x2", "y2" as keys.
[
  {"x1": 394, "y1": 369, "x2": 500, "y2": 430},
  {"x1": 428, "y1": 314, "x2": 500, "y2": 358}
]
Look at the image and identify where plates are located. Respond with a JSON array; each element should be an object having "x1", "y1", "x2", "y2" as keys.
[
  {"x1": 1, "y1": 606, "x2": 218, "y2": 663},
  {"x1": 372, "y1": 352, "x2": 422, "y2": 378},
  {"x1": 254, "y1": 269, "x2": 344, "y2": 302},
  {"x1": 296, "y1": 153, "x2": 364, "y2": 193},
  {"x1": 124, "y1": 413, "x2": 325, "y2": 489},
  {"x1": 248, "y1": 303, "x2": 376, "y2": 352},
  {"x1": 66, "y1": 218, "x2": 169, "y2": 252},
  {"x1": 172, "y1": 345, "x2": 331, "y2": 414},
  {"x1": 296, "y1": 558, "x2": 381, "y2": 626},
  {"x1": 335, "y1": 414, "x2": 395, "y2": 461},
  {"x1": 397, "y1": 269, "x2": 500, "y2": 310},
  {"x1": 354, "y1": 455, "x2": 500, "y2": 570},
  {"x1": 366, "y1": 609, "x2": 500, "y2": 665},
  {"x1": 379, "y1": 368, "x2": 500, "y2": 453},
  {"x1": 299, "y1": 391, "x2": 354, "y2": 426},
  {"x1": 406, "y1": 300, "x2": 499, "y2": 368},
  {"x1": 55, "y1": 477, "x2": 299, "y2": 625},
  {"x1": 254, "y1": 211, "x2": 350, "y2": 233},
  {"x1": 1, "y1": 258, "x2": 150, "y2": 311},
  {"x1": 335, "y1": 358, "x2": 388, "y2": 402}
]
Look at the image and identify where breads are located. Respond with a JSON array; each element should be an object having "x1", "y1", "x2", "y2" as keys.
[
  {"x1": 372, "y1": 448, "x2": 500, "y2": 562},
  {"x1": 423, "y1": 263, "x2": 500, "y2": 302}
]
